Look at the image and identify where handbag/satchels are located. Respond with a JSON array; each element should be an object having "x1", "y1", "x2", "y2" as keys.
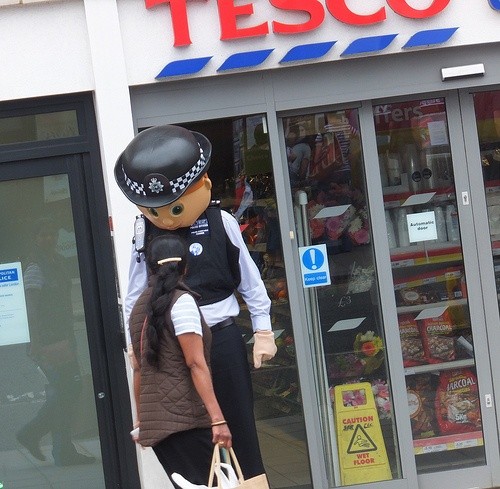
[{"x1": 207, "y1": 441, "x2": 269, "y2": 489}]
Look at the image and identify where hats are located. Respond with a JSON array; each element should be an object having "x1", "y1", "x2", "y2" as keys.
[{"x1": 113, "y1": 124, "x2": 212, "y2": 207}]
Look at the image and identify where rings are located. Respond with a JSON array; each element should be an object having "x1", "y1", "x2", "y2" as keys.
[{"x1": 218, "y1": 440, "x2": 225, "y2": 446}]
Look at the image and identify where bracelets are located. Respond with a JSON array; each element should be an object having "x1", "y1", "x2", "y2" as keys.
[{"x1": 210, "y1": 420, "x2": 229, "y2": 426}]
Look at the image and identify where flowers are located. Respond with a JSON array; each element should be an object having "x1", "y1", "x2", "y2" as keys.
[{"x1": 309, "y1": 204, "x2": 393, "y2": 419}]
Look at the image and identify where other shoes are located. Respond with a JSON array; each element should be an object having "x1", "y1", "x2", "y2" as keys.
[
  {"x1": 54, "y1": 445, "x2": 95, "y2": 465},
  {"x1": 14, "y1": 430, "x2": 49, "y2": 462}
]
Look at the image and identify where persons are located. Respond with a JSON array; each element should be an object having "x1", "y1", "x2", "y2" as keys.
[
  {"x1": 241, "y1": 103, "x2": 424, "y2": 256},
  {"x1": 14, "y1": 218, "x2": 95, "y2": 465},
  {"x1": 129, "y1": 236, "x2": 233, "y2": 489},
  {"x1": 114, "y1": 125, "x2": 279, "y2": 489}
]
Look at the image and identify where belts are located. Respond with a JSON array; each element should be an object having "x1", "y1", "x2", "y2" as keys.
[{"x1": 209, "y1": 316, "x2": 236, "y2": 333}]
